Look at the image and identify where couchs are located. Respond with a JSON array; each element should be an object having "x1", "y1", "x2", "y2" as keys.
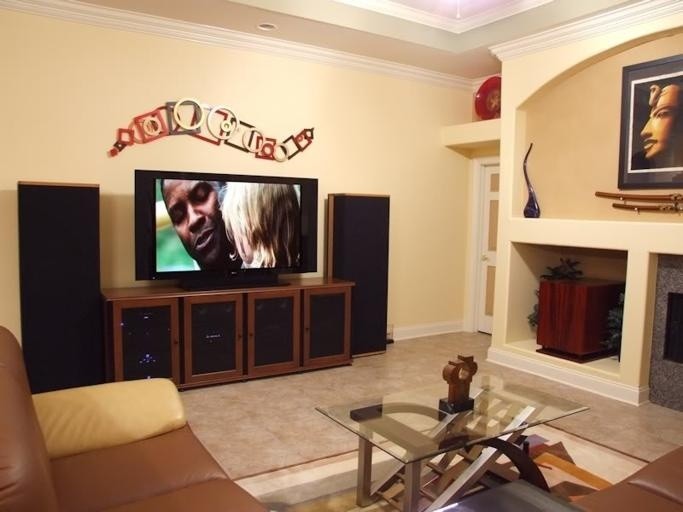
[
  {"x1": 437, "y1": 444, "x2": 681, "y2": 510},
  {"x1": 0, "y1": 324, "x2": 270, "y2": 509}
]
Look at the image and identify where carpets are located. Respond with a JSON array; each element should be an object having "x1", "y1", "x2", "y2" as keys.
[{"x1": 233, "y1": 412, "x2": 649, "y2": 512}]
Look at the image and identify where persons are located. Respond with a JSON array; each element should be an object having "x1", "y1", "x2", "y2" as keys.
[
  {"x1": 640, "y1": 83, "x2": 683, "y2": 169},
  {"x1": 160, "y1": 179, "x2": 242, "y2": 270},
  {"x1": 219, "y1": 182, "x2": 302, "y2": 269}
]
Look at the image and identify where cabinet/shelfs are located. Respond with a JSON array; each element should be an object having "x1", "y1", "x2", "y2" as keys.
[
  {"x1": 302, "y1": 276, "x2": 355, "y2": 374},
  {"x1": 180, "y1": 279, "x2": 301, "y2": 389},
  {"x1": 98, "y1": 286, "x2": 180, "y2": 391}
]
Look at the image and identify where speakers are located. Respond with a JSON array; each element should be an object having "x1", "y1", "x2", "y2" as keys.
[
  {"x1": 326, "y1": 193, "x2": 390, "y2": 358},
  {"x1": 17, "y1": 183, "x2": 107, "y2": 396}
]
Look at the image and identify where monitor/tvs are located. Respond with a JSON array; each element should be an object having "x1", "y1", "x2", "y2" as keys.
[{"x1": 134, "y1": 169, "x2": 319, "y2": 290}]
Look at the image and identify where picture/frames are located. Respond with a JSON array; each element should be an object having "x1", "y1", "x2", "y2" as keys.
[{"x1": 617, "y1": 52, "x2": 683, "y2": 188}]
